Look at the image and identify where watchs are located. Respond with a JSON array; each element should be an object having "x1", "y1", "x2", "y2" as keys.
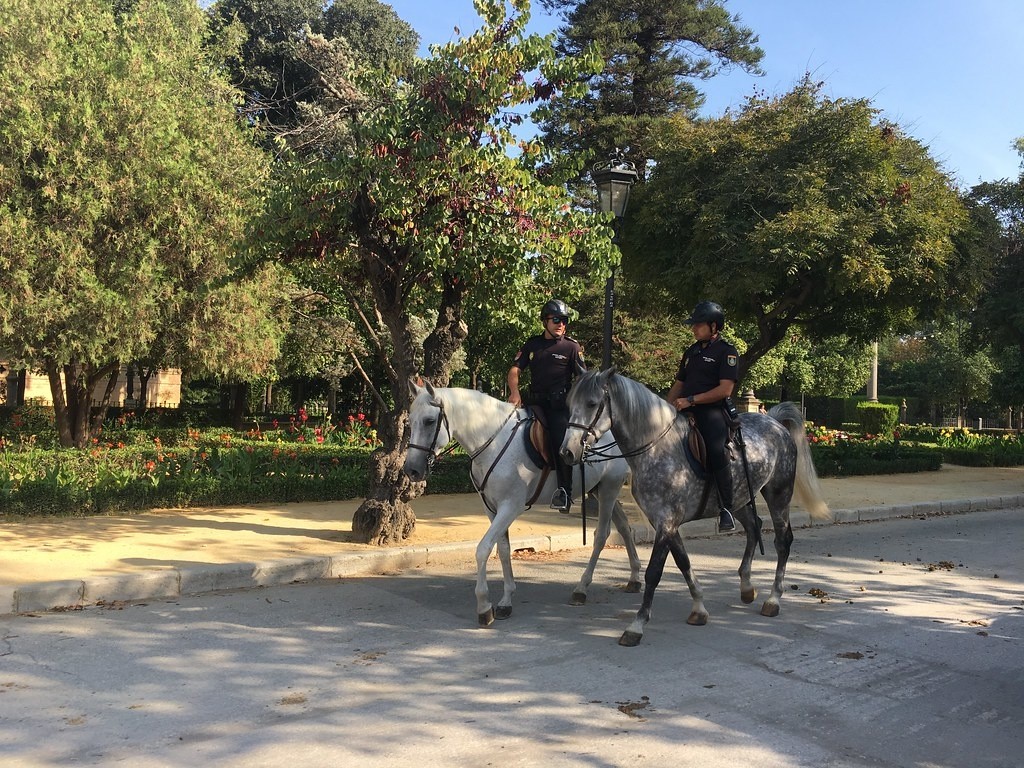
[{"x1": 688, "y1": 396, "x2": 694, "y2": 407}]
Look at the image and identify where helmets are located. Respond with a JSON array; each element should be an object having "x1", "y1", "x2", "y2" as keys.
[
  {"x1": 685, "y1": 301, "x2": 724, "y2": 331},
  {"x1": 540, "y1": 299, "x2": 569, "y2": 321}
]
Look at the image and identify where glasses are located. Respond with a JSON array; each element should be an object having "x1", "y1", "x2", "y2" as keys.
[{"x1": 544, "y1": 316, "x2": 568, "y2": 325}]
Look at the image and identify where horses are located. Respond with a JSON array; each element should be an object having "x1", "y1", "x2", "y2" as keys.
[
  {"x1": 404, "y1": 379, "x2": 642, "y2": 625},
  {"x1": 560, "y1": 360, "x2": 831, "y2": 647}
]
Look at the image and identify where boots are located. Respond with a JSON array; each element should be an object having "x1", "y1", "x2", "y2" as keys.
[
  {"x1": 553, "y1": 454, "x2": 574, "y2": 514},
  {"x1": 714, "y1": 463, "x2": 734, "y2": 532}
]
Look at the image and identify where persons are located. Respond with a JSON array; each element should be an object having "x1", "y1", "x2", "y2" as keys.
[
  {"x1": 506, "y1": 300, "x2": 587, "y2": 514},
  {"x1": 667, "y1": 301, "x2": 739, "y2": 530}
]
